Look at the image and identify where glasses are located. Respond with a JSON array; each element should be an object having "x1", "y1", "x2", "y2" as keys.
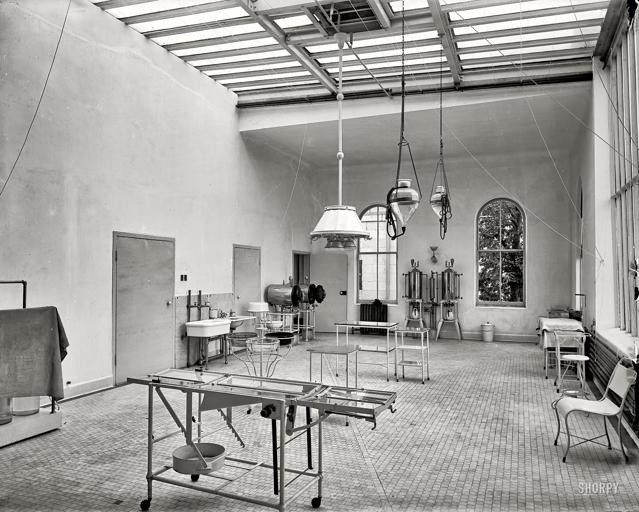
[{"x1": 308, "y1": 32, "x2": 370, "y2": 253}]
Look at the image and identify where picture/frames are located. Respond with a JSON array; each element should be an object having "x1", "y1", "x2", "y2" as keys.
[
  {"x1": 538, "y1": 317, "x2": 586, "y2": 380},
  {"x1": 307, "y1": 345, "x2": 360, "y2": 426}
]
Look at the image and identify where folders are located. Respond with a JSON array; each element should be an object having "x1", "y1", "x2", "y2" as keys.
[{"x1": 584, "y1": 327, "x2": 639, "y2": 428}]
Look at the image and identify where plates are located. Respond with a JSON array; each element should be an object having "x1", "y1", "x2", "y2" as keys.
[
  {"x1": 334, "y1": 320, "x2": 399, "y2": 382},
  {"x1": 394, "y1": 328, "x2": 431, "y2": 384}
]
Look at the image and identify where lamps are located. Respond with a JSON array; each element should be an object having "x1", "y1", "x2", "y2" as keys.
[
  {"x1": 553, "y1": 330, "x2": 590, "y2": 394},
  {"x1": 551, "y1": 357, "x2": 638, "y2": 463}
]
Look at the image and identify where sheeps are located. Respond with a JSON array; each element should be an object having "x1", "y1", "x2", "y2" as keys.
[
  {"x1": 229, "y1": 311, "x2": 237, "y2": 316},
  {"x1": 206, "y1": 304, "x2": 214, "y2": 312}
]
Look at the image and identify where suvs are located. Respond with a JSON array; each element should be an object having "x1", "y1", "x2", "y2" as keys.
[
  {"x1": 266, "y1": 284, "x2": 301, "y2": 306},
  {"x1": 480, "y1": 321, "x2": 496, "y2": 342},
  {"x1": 298, "y1": 284, "x2": 318, "y2": 304}
]
